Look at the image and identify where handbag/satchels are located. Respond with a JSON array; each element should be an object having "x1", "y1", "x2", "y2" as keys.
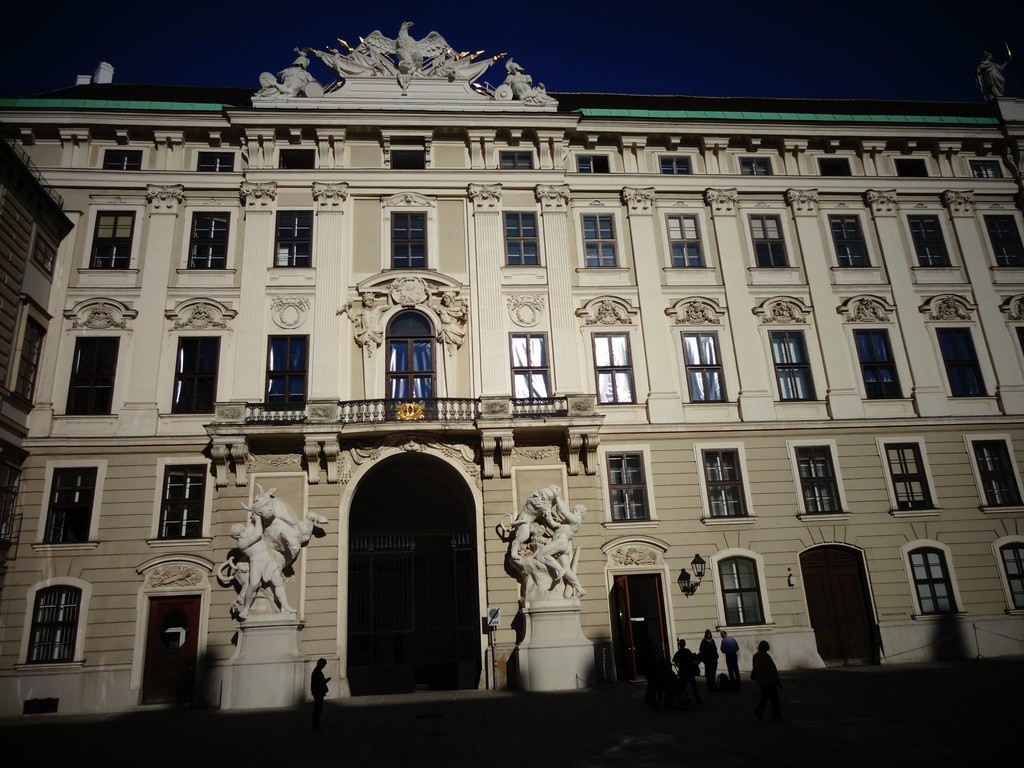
[{"x1": 751, "y1": 670, "x2": 759, "y2": 681}]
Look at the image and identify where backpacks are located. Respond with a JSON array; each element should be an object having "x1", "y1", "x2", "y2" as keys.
[{"x1": 717, "y1": 673, "x2": 730, "y2": 692}]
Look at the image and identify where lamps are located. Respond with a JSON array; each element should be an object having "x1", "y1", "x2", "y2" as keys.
[{"x1": 691, "y1": 553, "x2": 706, "y2": 586}]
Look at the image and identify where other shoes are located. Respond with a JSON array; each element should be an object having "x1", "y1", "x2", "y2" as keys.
[
  {"x1": 695, "y1": 700, "x2": 705, "y2": 706},
  {"x1": 771, "y1": 713, "x2": 784, "y2": 723},
  {"x1": 311, "y1": 721, "x2": 322, "y2": 731}
]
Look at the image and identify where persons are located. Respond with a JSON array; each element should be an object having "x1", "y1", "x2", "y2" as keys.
[
  {"x1": 344, "y1": 289, "x2": 392, "y2": 333},
  {"x1": 673, "y1": 630, "x2": 740, "y2": 705},
  {"x1": 311, "y1": 659, "x2": 330, "y2": 732},
  {"x1": 751, "y1": 642, "x2": 782, "y2": 720},
  {"x1": 534, "y1": 495, "x2": 588, "y2": 596},
  {"x1": 230, "y1": 513, "x2": 297, "y2": 619},
  {"x1": 425, "y1": 287, "x2": 466, "y2": 337}
]
